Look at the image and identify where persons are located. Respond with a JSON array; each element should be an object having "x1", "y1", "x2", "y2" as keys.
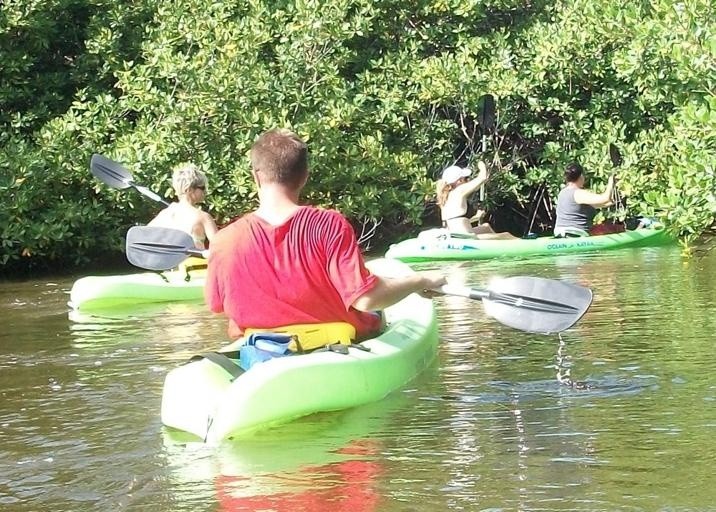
[
  {"x1": 145, "y1": 164, "x2": 219, "y2": 251},
  {"x1": 553, "y1": 163, "x2": 618, "y2": 239},
  {"x1": 434, "y1": 160, "x2": 520, "y2": 241},
  {"x1": 206, "y1": 130, "x2": 448, "y2": 352}
]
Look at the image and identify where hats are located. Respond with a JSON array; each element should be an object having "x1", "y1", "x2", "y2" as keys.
[{"x1": 442, "y1": 166, "x2": 472, "y2": 184}]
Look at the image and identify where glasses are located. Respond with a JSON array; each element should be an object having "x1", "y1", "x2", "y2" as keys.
[{"x1": 194, "y1": 186, "x2": 206, "y2": 191}]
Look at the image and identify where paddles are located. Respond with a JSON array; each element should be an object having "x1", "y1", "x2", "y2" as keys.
[
  {"x1": 477, "y1": 94, "x2": 494, "y2": 225},
  {"x1": 126, "y1": 227, "x2": 593, "y2": 334},
  {"x1": 90, "y1": 153, "x2": 171, "y2": 207},
  {"x1": 610, "y1": 144, "x2": 621, "y2": 199}
]
